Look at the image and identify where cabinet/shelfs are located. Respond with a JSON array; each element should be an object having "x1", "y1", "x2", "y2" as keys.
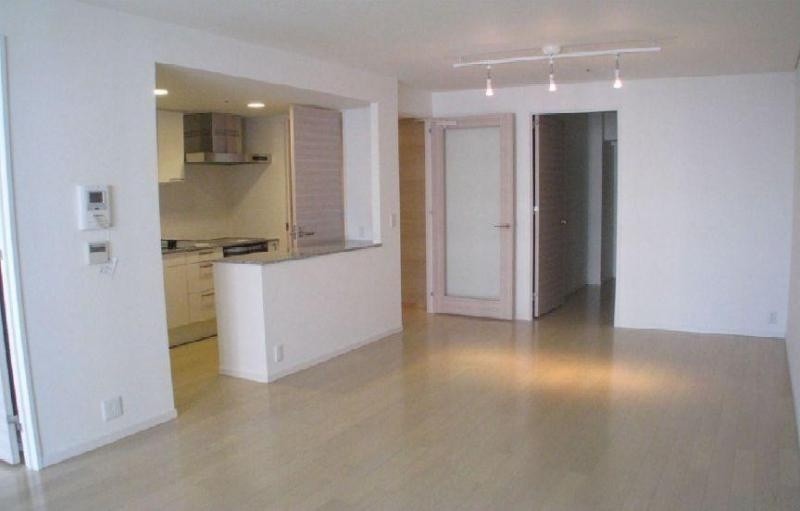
[
  {"x1": 157, "y1": 109, "x2": 184, "y2": 182},
  {"x1": 161, "y1": 247, "x2": 223, "y2": 349}
]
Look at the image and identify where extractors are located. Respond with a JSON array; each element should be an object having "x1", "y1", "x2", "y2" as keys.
[{"x1": 183, "y1": 111, "x2": 273, "y2": 166}]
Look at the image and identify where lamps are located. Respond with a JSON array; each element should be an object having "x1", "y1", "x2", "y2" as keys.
[{"x1": 452, "y1": 45, "x2": 660, "y2": 97}]
